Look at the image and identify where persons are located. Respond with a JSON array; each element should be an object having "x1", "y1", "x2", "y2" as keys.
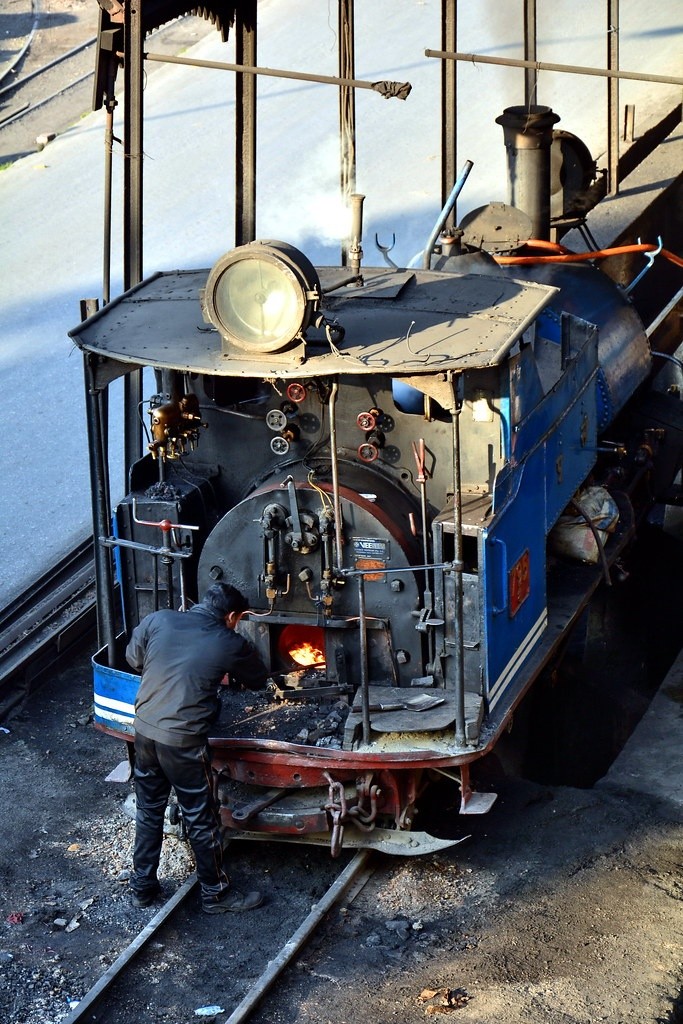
[{"x1": 125, "y1": 582, "x2": 268, "y2": 914}]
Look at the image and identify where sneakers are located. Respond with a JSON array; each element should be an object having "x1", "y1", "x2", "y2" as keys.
[
  {"x1": 202, "y1": 881, "x2": 264, "y2": 914},
  {"x1": 131, "y1": 886, "x2": 164, "y2": 907}
]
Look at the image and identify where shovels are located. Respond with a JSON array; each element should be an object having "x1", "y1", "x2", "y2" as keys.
[{"x1": 350, "y1": 694, "x2": 444, "y2": 713}]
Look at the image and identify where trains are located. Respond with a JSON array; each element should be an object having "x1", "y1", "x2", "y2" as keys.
[{"x1": 64, "y1": 0, "x2": 683, "y2": 862}]
